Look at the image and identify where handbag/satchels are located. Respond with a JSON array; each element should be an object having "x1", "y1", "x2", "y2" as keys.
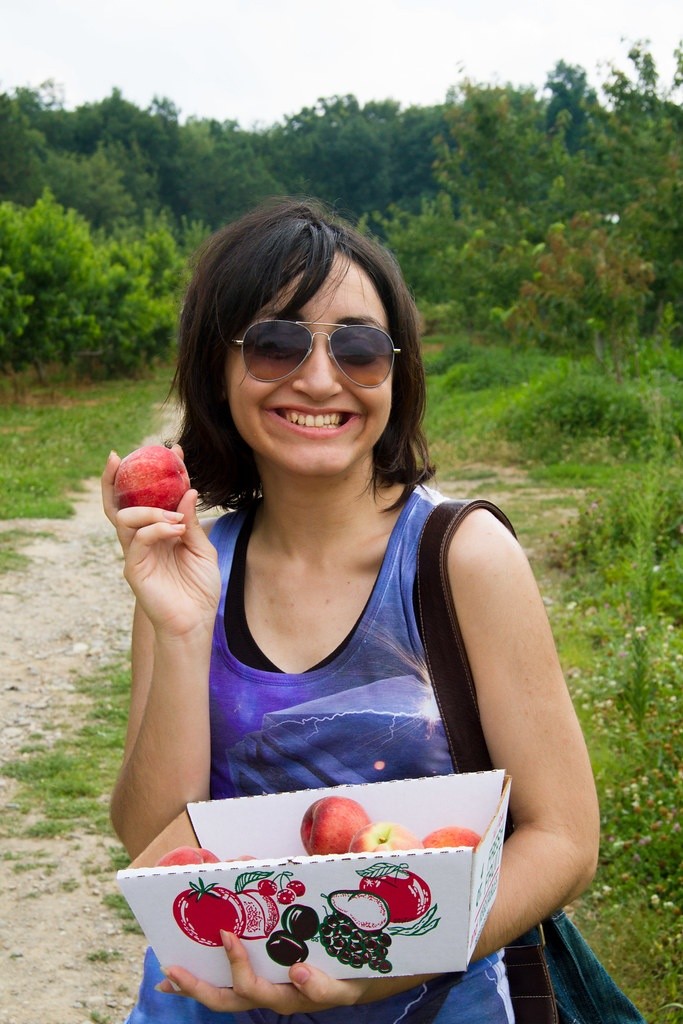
[{"x1": 515, "y1": 902, "x2": 646, "y2": 1023}]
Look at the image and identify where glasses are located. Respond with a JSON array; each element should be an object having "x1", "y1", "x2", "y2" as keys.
[{"x1": 228, "y1": 320, "x2": 404, "y2": 391}]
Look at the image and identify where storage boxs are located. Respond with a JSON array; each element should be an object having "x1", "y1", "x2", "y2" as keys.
[{"x1": 117, "y1": 769, "x2": 512, "y2": 992}]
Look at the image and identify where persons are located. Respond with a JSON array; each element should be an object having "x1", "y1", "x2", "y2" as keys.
[{"x1": 99, "y1": 196, "x2": 599, "y2": 1024}]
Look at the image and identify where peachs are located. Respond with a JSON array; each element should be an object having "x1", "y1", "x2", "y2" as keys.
[
  {"x1": 113, "y1": 444, "x2": 192, "y2": 511},
  {"x1": 154, "y1": 795, "x2": 482, "y2": 866}
]
